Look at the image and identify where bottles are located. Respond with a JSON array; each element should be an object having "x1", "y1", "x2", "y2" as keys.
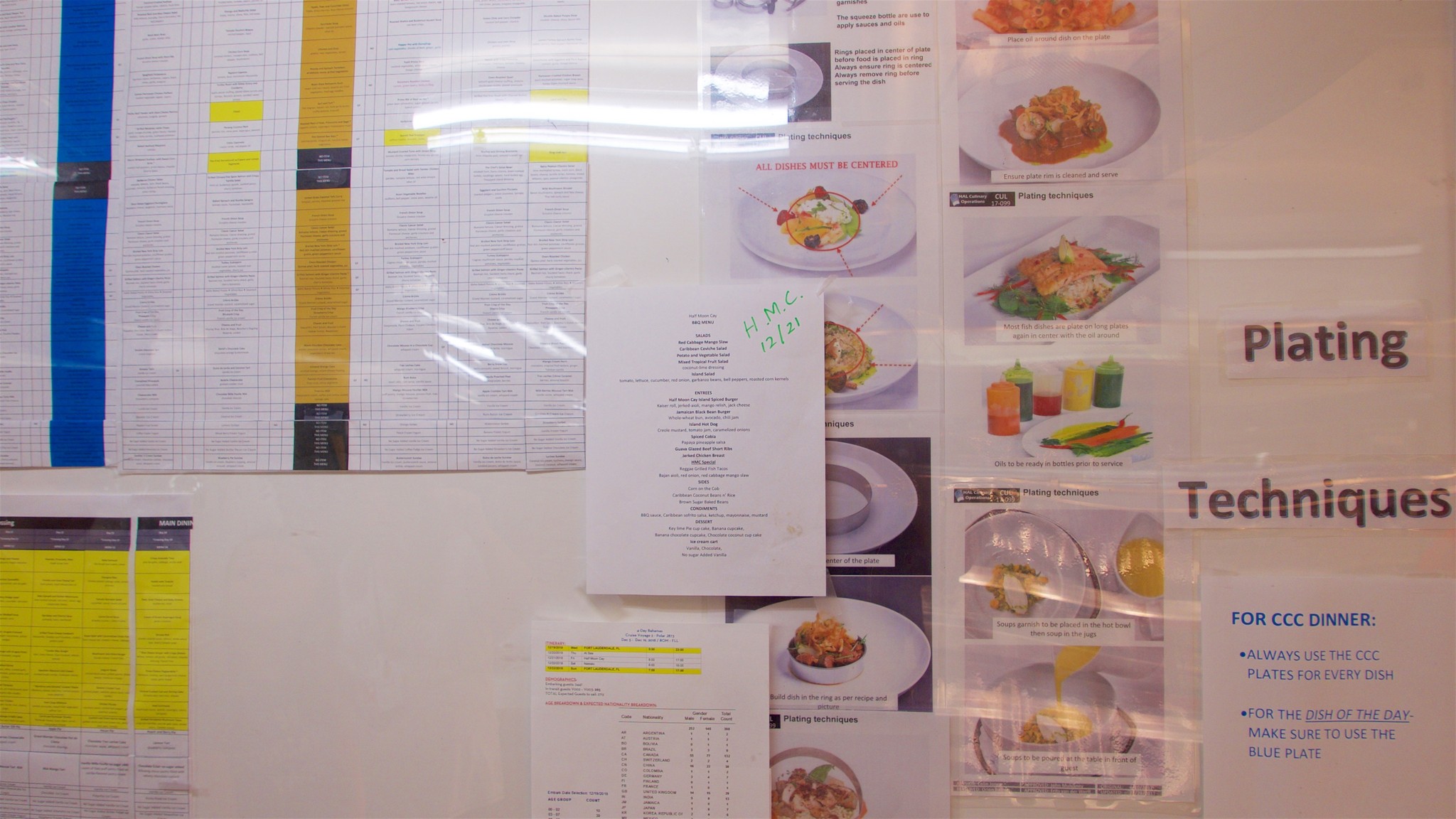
[
  {"x1": 1002, "y1": 359, "x2": 1034, "y2": 423},
  {"x1": 1093, "y1": 353, "x2": 1125, "y2": 409},
  {"x1": 1063, "y1": 359, "x2": 1094, "y2": 412},
  {"x1": 986, "y1": 374, "x2": 1021, "y2": 436}
]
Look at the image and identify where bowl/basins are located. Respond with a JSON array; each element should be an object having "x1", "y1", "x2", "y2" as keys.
[
  {"x1": 787, "y1": 636, "x2": 867, "y2": 685},
  {"x1": 1114, "y1": 511, "x2": 1163, "y2": 606},
  {"x1": 741, "y1": 58, "x2": 797, "y2": 99},
  {"x1": 826, "y1": 463, "x2": 873, "y2": 536},
  {"x1": 979, "y1": 662, "x2": 1118, "y2": 752}
]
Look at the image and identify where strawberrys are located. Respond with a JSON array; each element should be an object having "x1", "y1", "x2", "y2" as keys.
[
  {"x1": 814, "y1": 186, "x2": 831, "y2": 200},
  {"x1": 777, "y1": 210, "x2": 796, "y2": 225}
]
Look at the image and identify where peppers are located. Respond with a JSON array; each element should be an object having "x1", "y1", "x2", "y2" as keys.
[{"x1": 1039, "y1": 412, "x2": 1154, "y2": 457}]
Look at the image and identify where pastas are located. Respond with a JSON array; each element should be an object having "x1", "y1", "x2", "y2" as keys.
[{"x1": 973, "y1": 0, "x2": 1136, "y2": 33}]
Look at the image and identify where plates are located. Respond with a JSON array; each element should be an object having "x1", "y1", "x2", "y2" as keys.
[
  {"x1": 735, "y1": 596, "x2": 932, "y2": 697},
  {"x1": 955, "y1": 0, "x2": 1159, "y2": 48},
  {"x1": 958, "y1": 59, "x2": 1163, "y2": 170},
  {"x1": 1021, "y1": 405, "x2": 1152, "y2": 458},
  {"x1": 965, "y1": 513, "x2": 1085, "y2": 639},
  {"x1": 732, "y1": 169, "x2": 917, "y2": 272},
  {"x1": 974, "y1": 708, "x2": 1136, "y2": 791},
  {"x1": 964, "y1": 215, "x2": 1161, "y2": 346},
  {"x1": 825, "y1": 440, "x2": 919, "y2": 554},
  {"x1": 714, "y1": 46, "x2": 824, "y2": 109},
  {"x1": 824, "y1": 292, "x2": 918, "y2": 404}
]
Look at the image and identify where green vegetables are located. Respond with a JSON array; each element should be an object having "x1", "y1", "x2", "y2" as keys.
[
  {"x1": 809, "y1": 764, "x2": 833, "y2": 783},
  {"x1": 787, "y1": 629, "x2": 868, "y2": 661},
  {"x1": 992, "y1": 249, "x2": 1144, "y2": 320}
]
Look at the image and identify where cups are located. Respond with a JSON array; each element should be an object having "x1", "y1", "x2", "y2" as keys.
[{"x1": 1032, "y1": 362, "x2": 1064, "y2": 416}]
